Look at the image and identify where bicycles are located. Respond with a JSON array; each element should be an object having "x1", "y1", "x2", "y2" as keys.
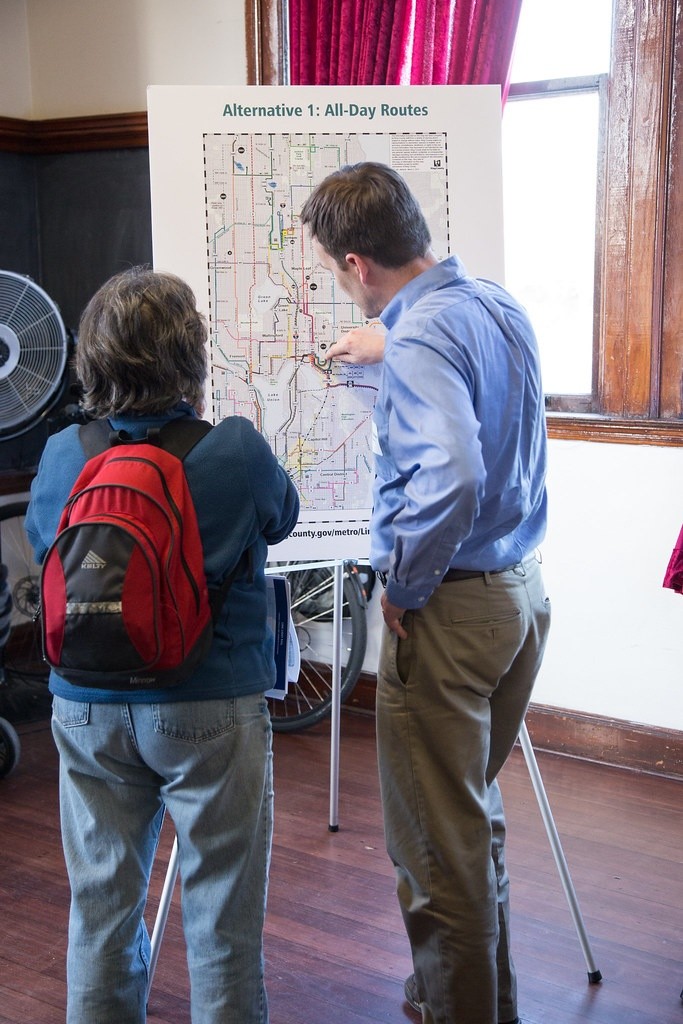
[{"x1": 0, "y1": 500, "x2": 369, "y2": 736}]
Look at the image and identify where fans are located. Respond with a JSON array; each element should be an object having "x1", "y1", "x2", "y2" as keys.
[{"x1": 1, "y1": 270, "x2": 80, "y2": 443}]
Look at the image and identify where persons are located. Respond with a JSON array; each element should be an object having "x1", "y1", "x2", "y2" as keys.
[
  {"x1": 24, "y1": 270, "x2": 299, "y2": 1024},
  {"x1": 301, "y1": 160, "x2": 552, "y2": 1024}
]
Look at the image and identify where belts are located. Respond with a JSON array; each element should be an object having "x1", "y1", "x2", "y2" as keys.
[{"x1": 380, "y1": 570, "x2": 519, "y2": 588}]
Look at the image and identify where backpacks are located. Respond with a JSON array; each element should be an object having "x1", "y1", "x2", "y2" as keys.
[{"x1": 38, "y1": 416, "x2": 250, "y2": 692}]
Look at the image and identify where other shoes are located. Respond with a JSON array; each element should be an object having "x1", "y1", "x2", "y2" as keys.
[{"x1": 404, "y1": 973, "x2": 522, "y2": 1024}]
